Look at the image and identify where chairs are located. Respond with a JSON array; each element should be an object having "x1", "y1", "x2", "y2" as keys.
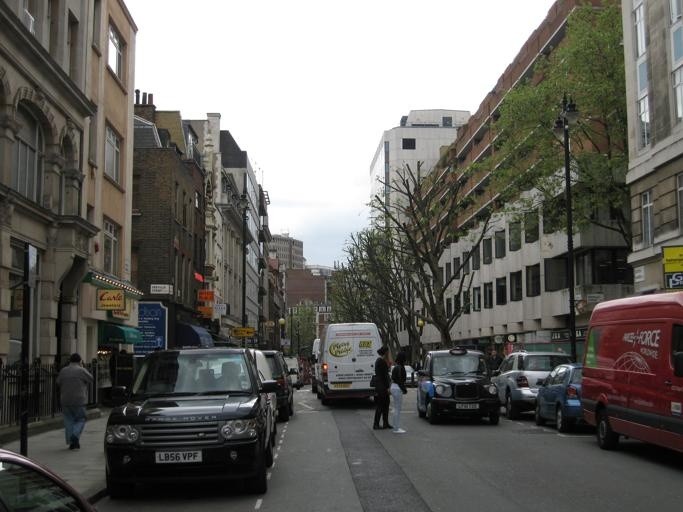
[{"x1": 196, "y1": 362, "x2": 242, "y2": 391}]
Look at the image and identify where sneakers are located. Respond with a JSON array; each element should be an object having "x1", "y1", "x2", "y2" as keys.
[{"x1": 391, "y1": 425, "x2": 406, "y2": 433}]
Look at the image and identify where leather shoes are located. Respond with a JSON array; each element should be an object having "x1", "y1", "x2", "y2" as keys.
[{"x1": 68, "y1": 433, "x2": 80, "y2": 450}]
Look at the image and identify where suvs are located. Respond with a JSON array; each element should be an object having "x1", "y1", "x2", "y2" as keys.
[
  {"x1": 100, "y1": 346, "x2": 307, "y2": 497},
  {"x1": 490, "y1": 350, "x2": 578, "y2": 420}
]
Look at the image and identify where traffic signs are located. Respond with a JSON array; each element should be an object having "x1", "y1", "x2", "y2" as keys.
[{"x1": 231, "y1": 327, "x2": 255, "y2": 338}]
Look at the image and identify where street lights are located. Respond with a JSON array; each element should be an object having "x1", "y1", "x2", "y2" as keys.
[
  {"x1": 237, "y1": 172, "x2": 253, "y2": 349},
  {"x1": 277, "y1": 318, "x2": 287, "y2": 353},
  {"x1": 417, "y1": 320, "x2": 424, "y2": 370},
  {"x1": 562, "y1": 88, "x2": 585, "y2": 365}
]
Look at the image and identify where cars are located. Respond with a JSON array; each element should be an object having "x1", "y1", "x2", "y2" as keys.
[
  {"x1": 415, "y1": 345, "x2": 502, "y2": 425},
  {"x1": 533, "y1": 362, "x2": 594, "y2": 434},
  {"x1": 0, "y1": 447, "x2": 98, "y2": 511},
  {"x1": 388, "y1": 363, "x2": 420, "y2": 386}
]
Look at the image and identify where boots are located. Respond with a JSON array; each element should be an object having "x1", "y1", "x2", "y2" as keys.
[{"x1": 373, "y1": 421, "x2": 393, "y2": 429}]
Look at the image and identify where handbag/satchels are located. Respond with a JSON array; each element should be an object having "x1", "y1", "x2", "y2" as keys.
[{"x1": 369, "y1": 375, "x2": 391, "y2": 388}]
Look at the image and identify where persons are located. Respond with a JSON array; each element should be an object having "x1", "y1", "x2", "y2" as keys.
[
  {"x1": 56, "y1": 353, "x2": 94, "y2": 449},
  {"x1": 374, "y1": 346, "x2": 408, "y2": 434}
]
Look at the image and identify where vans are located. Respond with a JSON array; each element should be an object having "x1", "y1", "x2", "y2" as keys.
[
  {"x1": 311, "y1": 320, "x2": 392, "y2": 407},
  {"x1": 578, "y1": 290, "x2": 682, "y2": 460}
]
